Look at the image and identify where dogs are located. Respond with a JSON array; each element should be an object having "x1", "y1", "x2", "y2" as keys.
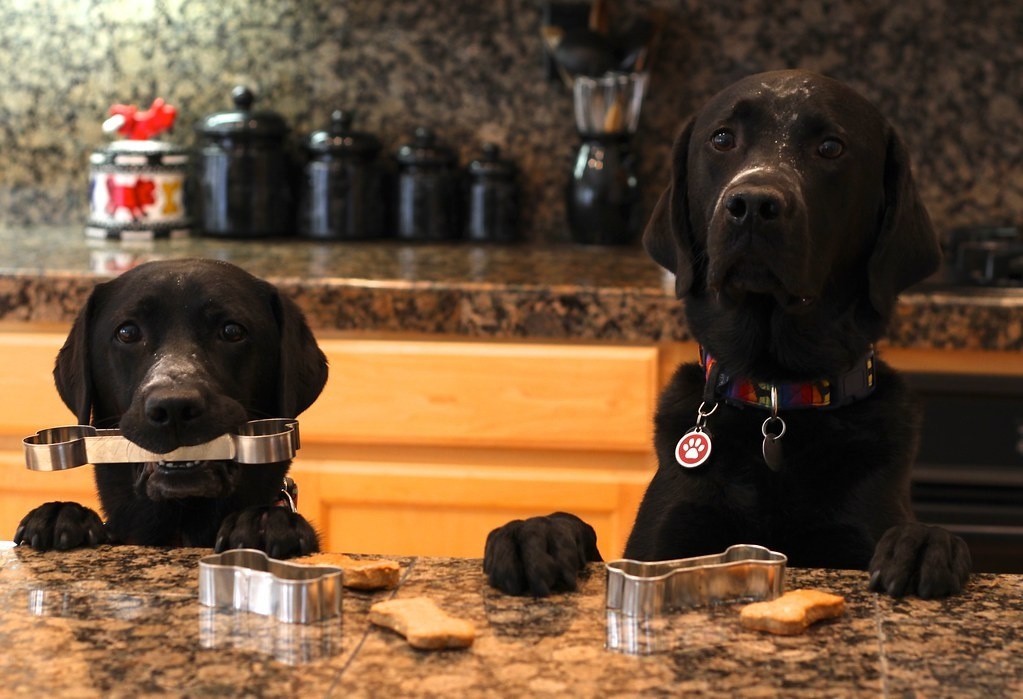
[
  {"x1": 484, "y1": 69, "x2": 971, "y2": 601},
  {"x1": 13, "y1": 255, "x2": 327, "y2": 562}
]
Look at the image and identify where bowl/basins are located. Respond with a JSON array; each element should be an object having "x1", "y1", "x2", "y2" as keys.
[{"x1": 84, "y1": 138, "x2": 193, "y2": 242}]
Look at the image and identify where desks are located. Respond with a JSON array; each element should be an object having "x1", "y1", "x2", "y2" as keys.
[{"x1": 0, "y1": 541, "x2": 1023, "y2": 699}]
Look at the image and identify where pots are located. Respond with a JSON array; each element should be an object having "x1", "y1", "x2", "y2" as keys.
[{"x1": 940, "y1": 215, "x2": 1023, "y2": 288}]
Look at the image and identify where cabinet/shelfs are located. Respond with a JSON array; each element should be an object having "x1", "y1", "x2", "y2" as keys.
[{"x1": 0, "y1": 332, "x2": 665, "y2": 562}]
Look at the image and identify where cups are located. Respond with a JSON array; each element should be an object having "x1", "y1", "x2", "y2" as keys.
[
  {"x1": 459, "y1": 140, "x2": 529, "y2": 243},
  {"x1": 387, "y1": 126, "x2": 459, "y2": 242},
  {"x1": 194, "y1": 87, "x2": 293, "y2": 237},
  {"x1": 297, "y1": 110, "x2": 383, "y2": 240}
]
